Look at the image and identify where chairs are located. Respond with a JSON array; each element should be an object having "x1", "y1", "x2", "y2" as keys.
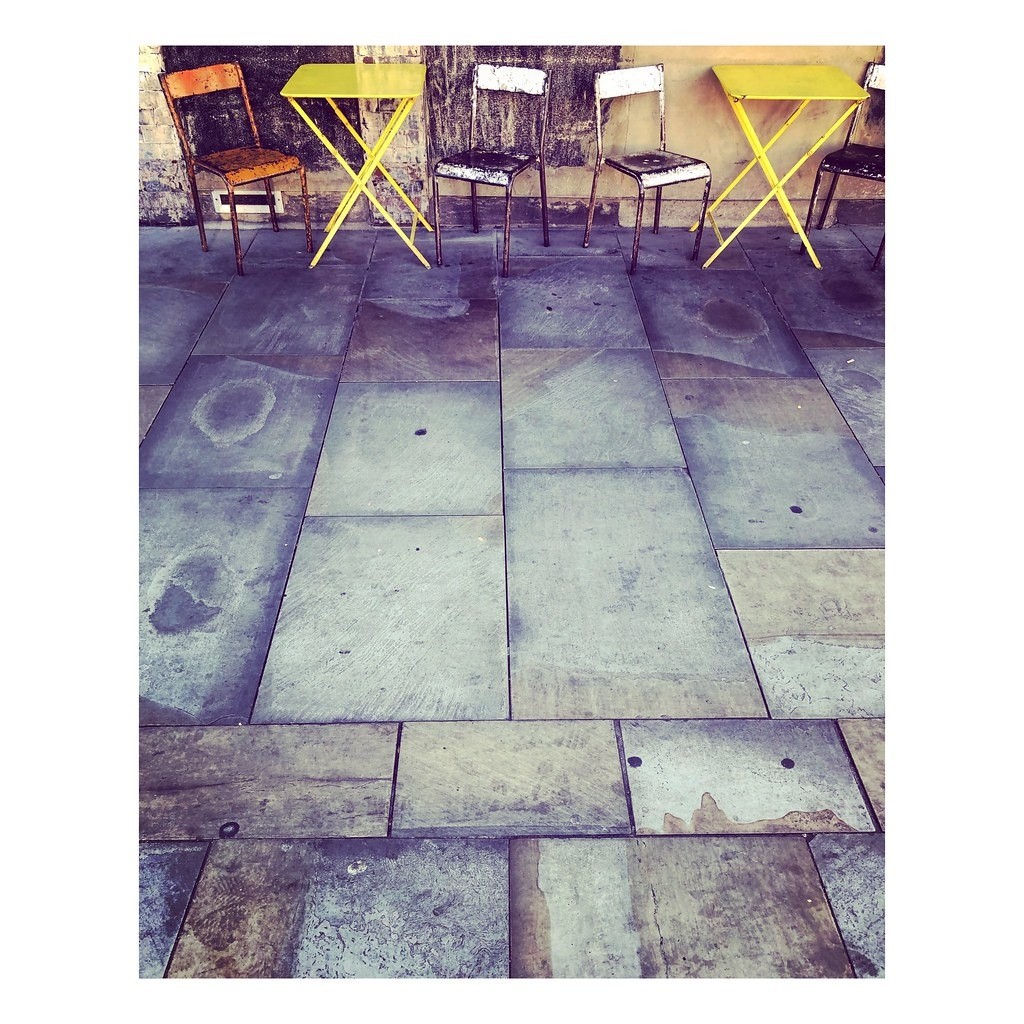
[
  {"x1": 157, "y1": 60, "x2": 314, "y2": 277},
  {"x1": 432, "y1": 64, "x2": 554, "y2": 278},
  {"x1": 583, "y1": 63, "x2": 711, "y2": 275},
  {"x1": 800, "y1": 61, "x2": 885, "y2": 272}
]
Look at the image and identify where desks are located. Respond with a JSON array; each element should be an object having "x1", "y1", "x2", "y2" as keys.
[
  {"x1": 279, "y1": 64, "x2": 434, "y2": 272},
  {"x1": 688, "y1": 63, "x2": 871, "y2": 270}
]
